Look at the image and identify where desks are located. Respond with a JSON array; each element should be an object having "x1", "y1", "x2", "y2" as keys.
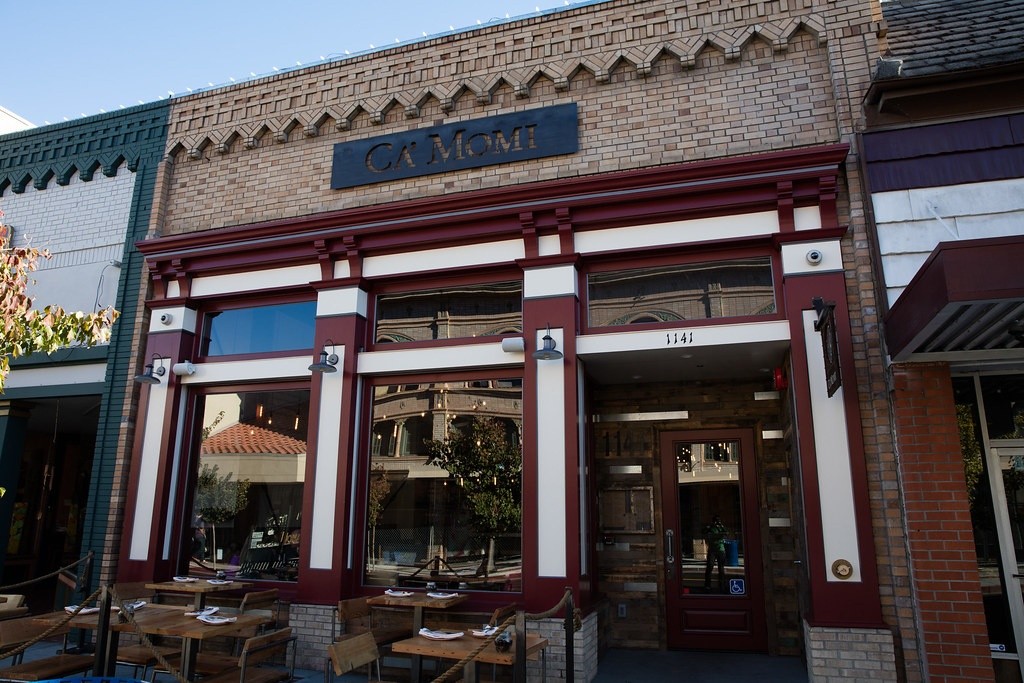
[
  {"x1": 144, "y1": 578, "x2": 253, "y2": 658},
  {"x1": 392, "y1": 630, "x2": 548, "y2": 683},
  {"x1": 32, "y1": 606, "x2": 268, "y2": 683},
  {"x1": 366, "y1": 592, "x2": 468, "y2": 683}
]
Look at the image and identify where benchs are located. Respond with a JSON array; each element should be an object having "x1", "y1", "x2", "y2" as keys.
[{"x1": 0, "y1": 611, "x2": 94, "y2": 683}]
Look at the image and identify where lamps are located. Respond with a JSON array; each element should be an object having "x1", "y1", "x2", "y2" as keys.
[
  {"x1": 133, "y1": 352, "x2": 165, "y2": 384},
  {"x1": 532, "y1": 322, "x2": 563, "y2": 361},
  {"x1": 308, "y1": 339, "x2": 339, "y2": 373}
]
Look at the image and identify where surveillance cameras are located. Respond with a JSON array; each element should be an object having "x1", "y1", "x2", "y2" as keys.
[
  {"x1": 160, "y1": 313, "x2": 173, "y2": 324},
  {"x1": 806, "y1": 249, "x2": 823, "y2": 263}
]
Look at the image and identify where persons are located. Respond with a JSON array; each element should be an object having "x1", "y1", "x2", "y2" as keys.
[{"x1": 702, "y1": 514, "x2": 729, "y2": 590}]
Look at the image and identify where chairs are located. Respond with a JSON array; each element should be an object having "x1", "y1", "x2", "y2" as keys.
[
  {"x1": 326, "y1": 630, "x2": 382, "y2": 683},
  {"x1": 112, "y1": 581, "x2": 184, "y2": 648},
  {"x1": 204, "y1": 588, "x2": 285, "y2": 659},
  {"x1": 150, "y1": 624, "x2": 260, "y2": 683},
  {"x1": 84, "y1": 644, "x2": 182, "y2": 681},
  {"x1": 329, "y1": 597, "x2": 408, "y2": 683},
  {"x1": 199, "y1": 627, "x2": 299, "y2": 683}
]
